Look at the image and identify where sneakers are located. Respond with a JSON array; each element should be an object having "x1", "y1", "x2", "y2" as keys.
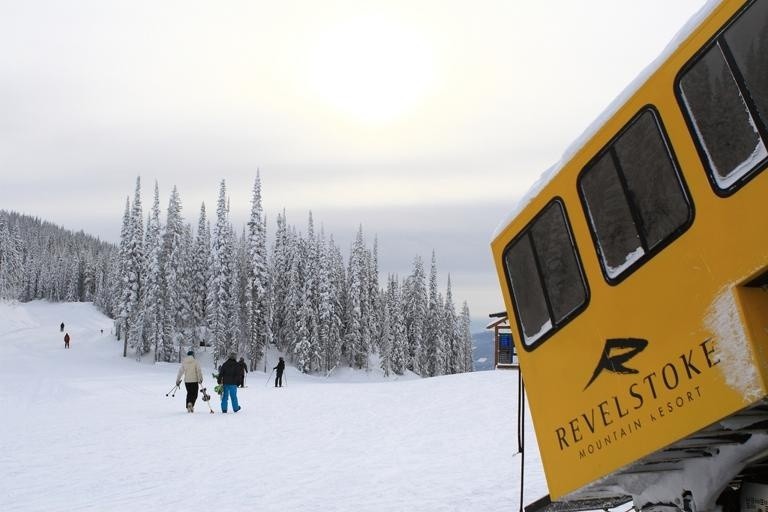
[
  {"x1": 235, "y1": 406, "x2": 240, "y2": 412},
  {"x1": 223, "y1": 410, "x2": 227, "y2": 413},
  {"x1": 188, "y1": 403, "x2": 193, "y2": 413}
]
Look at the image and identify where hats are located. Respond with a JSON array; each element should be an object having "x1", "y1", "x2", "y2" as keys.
[
  {"x1": 229, "y1": 353, "x2": 237, "y2": 359},
  {"x1": 188, "y1": 351, "x2": 194, "y2": 356}
]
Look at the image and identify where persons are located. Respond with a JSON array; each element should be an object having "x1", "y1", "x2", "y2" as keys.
[
  {"x1": 217, "y1": 352, "x2": 241, "y2": 413},
  {"x1": 237, "y1": 357, "x2": 249, "y2": 388},
  {"x1": 60, "y1": 322, "x2": 65, "y2": 332},
  {"x1": 63, "y1": 332, "x2": 71, "y2": 349},
  {"x1": 175, "y1": 350, "x2": 204, "y2": 413},
  {"x1": 217, "y1": 364, "x2": 224, "y2": 401},
  {"x1": 272, "y1": 357, "x2": 285, "y2": 387}
]
